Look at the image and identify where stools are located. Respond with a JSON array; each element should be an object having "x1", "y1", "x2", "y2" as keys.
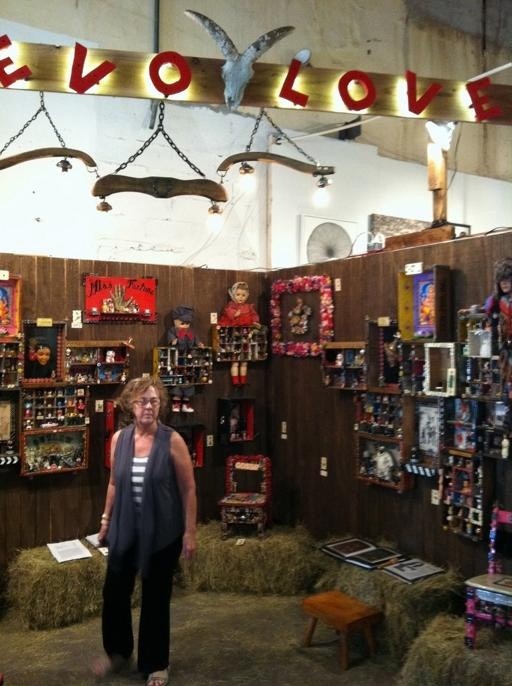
[{"x1": 300, "y1": 591, "x2": 379, "y2": 671}]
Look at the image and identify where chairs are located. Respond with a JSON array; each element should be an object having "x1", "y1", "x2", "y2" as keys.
[
  {"x1": 220, "y1": 455, "x2": 274, "y2": 535},
  {"x1": 464, "y1": 501, "x2": 512, "y2": 647}
]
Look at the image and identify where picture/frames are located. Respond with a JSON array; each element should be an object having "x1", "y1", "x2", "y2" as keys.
[
  {"x1": 447, "y1": 222, "x2": 472, "y2": 238},
  {"x1": 21, "y1": 317, "x2": 67, "y2": 389},
  {"x1": 298, "y1": 213, "x2": 361, "y2": 267},
  {"x1": 0, "y1": 272, "x2": 24, "y2": 345}
]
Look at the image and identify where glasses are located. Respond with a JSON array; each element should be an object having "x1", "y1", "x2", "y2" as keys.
[{"x1": 130, "y1": 398, "x2": 160, "y2": 407}]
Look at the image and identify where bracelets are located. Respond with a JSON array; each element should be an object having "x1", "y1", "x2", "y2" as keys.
[
  {"x1": 100, "y1": 519, "x2": 110, "y2": 525},
  {"x1": 102, "y1": 513, "x2": 112, "y2": 521}
]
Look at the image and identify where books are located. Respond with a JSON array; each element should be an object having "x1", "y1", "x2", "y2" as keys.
[
  {"x1": 319, "y1": 537, "x2": 409, "y2": 570},
  {"x1": 383, "y1": 558, "x2": 446, "y2": 585}
]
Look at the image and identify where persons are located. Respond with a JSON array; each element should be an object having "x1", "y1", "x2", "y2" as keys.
[
  {"x1": 25, "y1": 344, "x2": 56, "y2": 377},
  {"x1": 94, "y1": 372, "x2": 198, "y2": 686},
  {"x1": 109, "y1": 284, "x2": 133, "y2": 312},
  {"x1": 481, "y1": 260, "x2": 511, "y2": 352},
  {"x1": 374, "y1": 446, "x2": 393, "y2": 482},
  {"x1": 167, "y1": 304, "x2": 205, "y2": 413},
  {"x1": 216, "y1": 282, "x2": 260, "y2": 385}
]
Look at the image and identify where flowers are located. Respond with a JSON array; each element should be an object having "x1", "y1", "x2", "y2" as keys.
[{"x1": 269, "y1": 273, "x2": 334, "y2": 359}]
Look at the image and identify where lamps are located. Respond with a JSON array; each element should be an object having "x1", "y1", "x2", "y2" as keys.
[{"x1": 425, "y1": 116, "x2": 460, "y2": 152}]
